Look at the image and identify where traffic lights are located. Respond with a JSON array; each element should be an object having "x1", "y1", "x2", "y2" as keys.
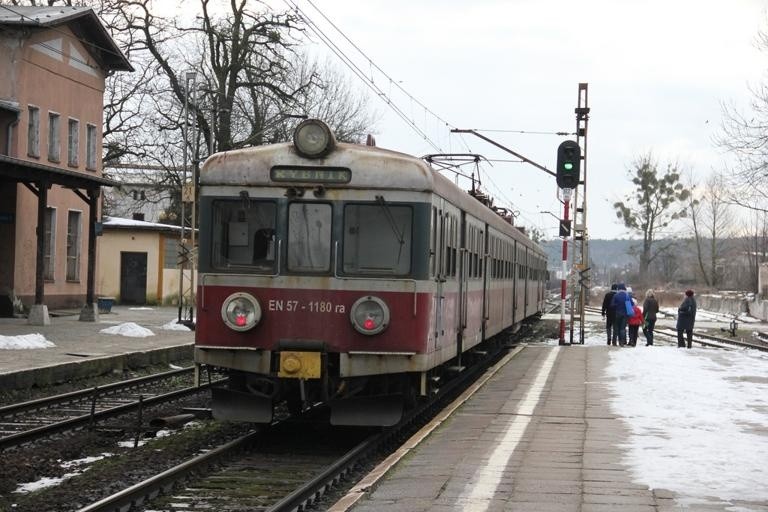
[{"x1": 557, "y1": 141, "x2": 580, "y2": 188}]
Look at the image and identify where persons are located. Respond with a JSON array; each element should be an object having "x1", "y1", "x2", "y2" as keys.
[
  {"x1": 675, "y1": 289, "x2": 696, "y2": 348},
  {"x1": 640, "y1": 289, "x2": 658, "y2": 346},
  {"x1": 627, "y1": 298, "x2": 644, "y2": 346},
  {"x1": 610, "y1": 282, "x2": 634, "y2": 347},
  {"x1": 600, "y1": 283, "x2": 619, "y2": 346}
]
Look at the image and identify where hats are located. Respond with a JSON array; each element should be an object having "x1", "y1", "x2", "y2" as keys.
[{"x1": 611, "y1": 283, "x2": 632, "y2": 292}]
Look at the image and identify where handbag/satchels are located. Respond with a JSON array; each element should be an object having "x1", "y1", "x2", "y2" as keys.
[
  {"x1": 678, "y1": 309, "x2": 692, "y2": 325},
  {"x1": 625, "y1": 301, "x2": 634, "y2": 316}
]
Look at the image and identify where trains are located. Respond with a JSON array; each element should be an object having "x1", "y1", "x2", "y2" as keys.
[{"x1": 194, "y1": 119, "x2": 545, "y2": 426}]
